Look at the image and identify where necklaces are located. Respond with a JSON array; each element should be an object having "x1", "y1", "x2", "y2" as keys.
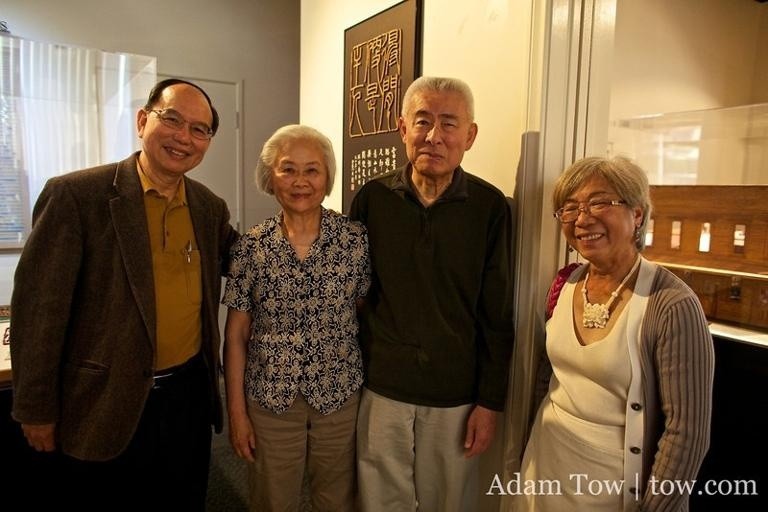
[{"x1": 577, "y1": 253, "x2": 644, "y2": 329}]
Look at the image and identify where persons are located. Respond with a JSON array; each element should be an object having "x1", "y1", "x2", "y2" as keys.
[
  {"x1": 222, "y1": 124, "x2": 372, "y2": 510},
  {"x1": 515, "y1": 153, "x2": 718, "y2": 510},
  {"x1": 347, "y1": 73, "x2": 513, "y2": 511},
  {"x1": 9, "y1": 79, "x2": 242, "y2": 509}
]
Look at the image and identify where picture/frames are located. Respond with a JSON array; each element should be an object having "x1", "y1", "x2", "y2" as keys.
[{"x1": 342, "y1": 0, "x2": 425, "y2": 215}]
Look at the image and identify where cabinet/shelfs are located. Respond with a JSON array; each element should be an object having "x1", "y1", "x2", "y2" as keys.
[{"x1": 651, "y1": 261, "x2": 768, "y2": 349}]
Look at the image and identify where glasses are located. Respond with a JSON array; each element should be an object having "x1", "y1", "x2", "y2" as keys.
[
  {"x1": 552, "y1": 198, "x2": 627, "y2": 223},
  {"x1": 150, "y1": 106, "x2": 213, "y2": 140}
]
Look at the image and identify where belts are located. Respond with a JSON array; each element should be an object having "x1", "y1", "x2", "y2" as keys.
[{"x1": 152, "y1": 371, "x2": 176, "y2": 392}]
